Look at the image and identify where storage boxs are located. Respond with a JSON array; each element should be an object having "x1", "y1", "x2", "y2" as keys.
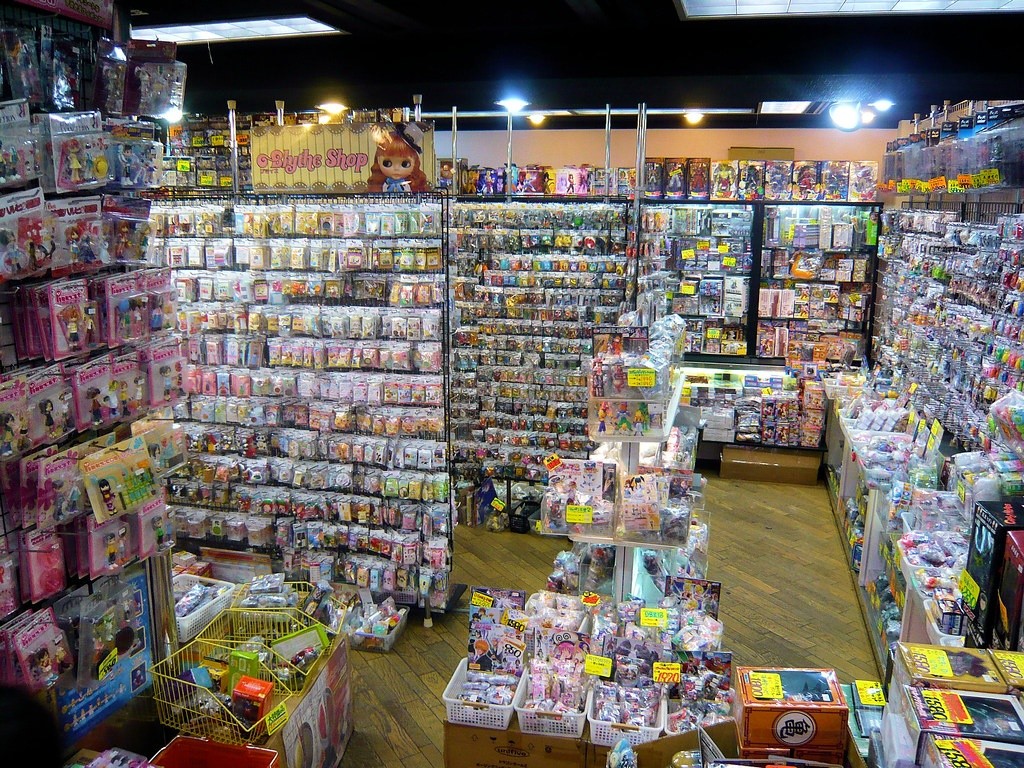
[
  {"x1": 719, "y1": 443, "x2": 823, "y2": 486},
  {"x1": 442, "y1": 666, "x2": 869, "y2": 768},
  {"x1": 824, "y1": 366, "x2": 1024, "y2": 767},
  {"x1": 436, "y1": 158, "x2": 878, "y2": 361}
]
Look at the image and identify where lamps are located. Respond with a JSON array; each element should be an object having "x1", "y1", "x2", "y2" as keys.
[{"x1": 828, "y1": 100, "x2": 862, "y2": 132}]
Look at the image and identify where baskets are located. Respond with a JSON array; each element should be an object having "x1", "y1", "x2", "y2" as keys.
[
  {"x1": 825, "y1": 375, "x2": 967, "y2": 647},
  {"x1": 443, "y1": 657, "x2": 527, "y2": 731},
  {"x1": 514, "y1": 667, "x2": 590, "y2": 739},
  {"x1": 230, "y1": 581, "x2": 347, "y2": 656},
  {"x1": 195, "y1": 608, "x2": 326, "y2": 697},
  {"x1": 170, "y1": 574, "x2": 235, "y2": 643},
  {"x1": 148, "y1": 640, "x2": 293, "y2": 745},
  {"x1": 664, "y1": 690, "x2": 678, "y2": 736},
  {"x1": 587, "y1": 685, "x2": 665, "y2": 748}
]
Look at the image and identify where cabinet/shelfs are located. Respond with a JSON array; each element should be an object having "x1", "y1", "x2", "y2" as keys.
[{"x1": 538, "y1": 372, "x2": 699, "y2": 607}]
